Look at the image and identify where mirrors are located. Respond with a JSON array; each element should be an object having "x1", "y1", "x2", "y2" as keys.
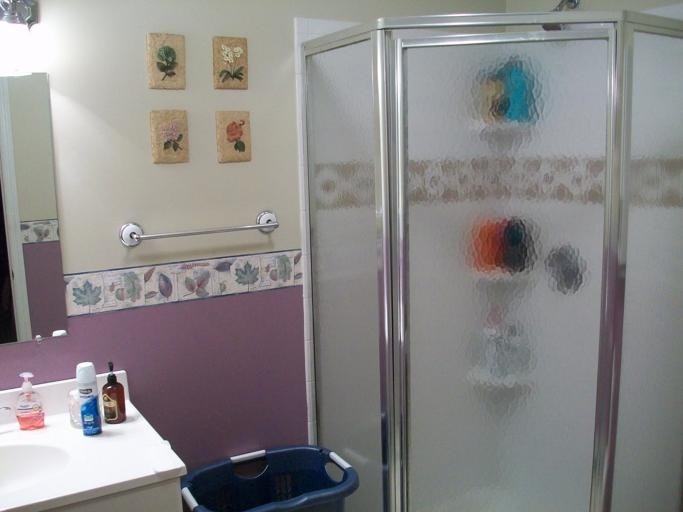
[{"x1": 0, "y1": 72, "x2": 69, "y2": 345}]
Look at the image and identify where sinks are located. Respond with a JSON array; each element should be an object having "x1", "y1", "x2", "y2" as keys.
[{"x1": 0, "y1": 444, "x2": 72, "y2": 496}]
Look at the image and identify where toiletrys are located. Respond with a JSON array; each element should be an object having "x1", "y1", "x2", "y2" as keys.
[
  {"x1": 75, "y1": 361, "x2": 101, "y2": 436},
  {"x1": 15, "y1": 372, "x2": 47, "y2": 430},
  {"x1": 67, "y1": 388, "x2": 83, "y2": 428}
]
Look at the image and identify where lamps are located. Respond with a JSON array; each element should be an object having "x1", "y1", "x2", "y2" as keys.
[{"x1": 0, "y1": 0, "x2": 60, "y2": 77}]
